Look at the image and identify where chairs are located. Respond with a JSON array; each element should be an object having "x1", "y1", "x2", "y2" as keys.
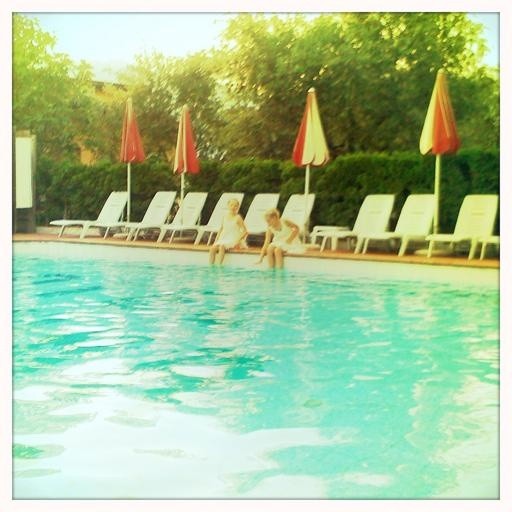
[
  {"x1": 125, "y1": 192, "x2": 208, "y2": 243},
  {"x1": 425, "y1": 195, "x2": 498, "y2": 261},
  {"x1": 80, "y1": 191, "x2": 178, "y2": 241},
  {"x1": 280, "y1": 194, "x2": 315, "y2": 243},
  {"x1": 201, "y1": 193, "x2": 280, "y2": 246},
  {"x1": 467, "y1": 235, "x2": 500, "y2": 261},
  {"x1": 354, "y1": 194, "x2": 437, "y2": 256},
  {"x1": 50, "y1": 191, "x2": 128, "y2": 239},
  {"x1": 309, "y1": 194, "x2": 395, "y2": 254},
  {"x1": 162, "y1": 192, "x2": 244, "y2": 247}
]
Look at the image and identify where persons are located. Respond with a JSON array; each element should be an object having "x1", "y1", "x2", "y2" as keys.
[
  {"x1": 207, "y1": 197, "x2": 248, "y2": 265},
  {"x1": 255, "y1": 206, "x2": 299, "y2": 270}
]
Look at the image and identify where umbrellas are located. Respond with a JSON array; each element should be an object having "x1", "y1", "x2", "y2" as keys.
[
  {"x1": 292, "y1": 86, "x2": 331, "y2": 243},
  {"x1": 419, "y1": 67, "x2": 462, "y2": 237},
  {"x1": 173, "y1": 102, "x2": 201, "y2": 224},
  {"x1": 121, "y1": 96, "x2": 147, "y2": 222}
]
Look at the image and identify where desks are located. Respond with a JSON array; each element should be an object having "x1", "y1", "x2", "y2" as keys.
[{"x1": 311, "y1": 226, "x2": 351, "y2": 253}]
